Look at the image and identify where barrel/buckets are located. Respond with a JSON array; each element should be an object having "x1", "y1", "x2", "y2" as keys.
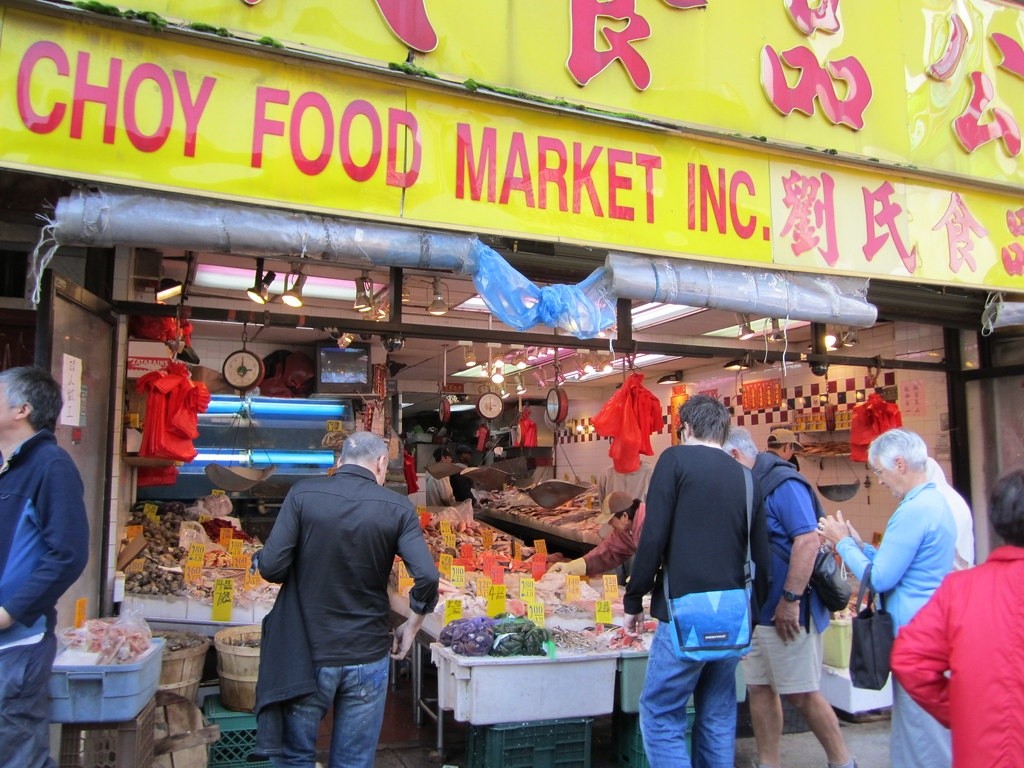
[
  {"x1": 214, "y1": 624, "x2": 262, "y2": 713},
  {"x1": 150, "y1": 628, "x2": 211, "y2": 706}
]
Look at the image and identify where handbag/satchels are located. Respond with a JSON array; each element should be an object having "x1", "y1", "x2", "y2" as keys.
[
  {"x1": 667, "y1": 588, "x2": 752, "y2": 662},
  {"x1": 849, "y1": 562, "x2": 893, "y2": 691},
  {"x1": 813, "y1": 552, "x2": 852, "y2": 612}
]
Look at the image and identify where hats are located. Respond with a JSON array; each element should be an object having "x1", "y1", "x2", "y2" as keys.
[
  {"x1": 433, "y1": 447, "x2": 453, "y2": 461},
  {"x1": 594, "y1": 491, "x2": 634, "y2": 524},
  {"x1": 767, "y1": 428, "x2": 804, "y2": 451}
]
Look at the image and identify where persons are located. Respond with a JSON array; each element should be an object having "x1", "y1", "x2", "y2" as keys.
[
  {"x1": 767, "y1": 428, "x2": 803, "y2": 462},
  {"x1": 599, "y1": 458, "x2": 655, "y2": 501},
  {"x1": 426, "y1": 448, "x2": 456, "y2": 508},
  {"x1": 547, "y1": 491, "x2": 647, "y2": 576},
  {"x1": 452, "y1": 446, "x2": 476, "y2": 508},
  {"x1": 249, "y1": 431, "x2": 439, "y2": 768},
  {"x1": 721, "y1": 427, "x2": 859, "y2": 768},
  {"x1": 889, "y1": 469, "x2": 1024, "y2": 768},
  {"x1": 0, "y1": 365, "x2": 89, "y2": 768},
  {"x1": 922, "y1": 457, "x2": 974, "y2": 572},
  {"x1": 816, "y1": 430, "x2": 958, "y2": 768},
  {"x1": 624, "y1": 396, "x2": 769, "y2": 768}
]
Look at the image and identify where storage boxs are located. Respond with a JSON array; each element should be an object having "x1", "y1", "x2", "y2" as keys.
[
  {"x1": 822, "y1": 619, "x2": 852, "y2": 668},
  {"x1": 116, "y1": 593, "x2": 272, "y2": 623},
  {"x1": 56, "y1": 697, "x2": 155, "y2": 768},
  {"x1": 463, "y1": 717, "x2": 591, "y2": 768},
  {"x1": 50, "y1": 636, "x2": 166, "y2": 726},
  {"x1": 622, "y1": 647, "x2": 749, "y2": 713},
  {"x1": 203, "y1": 693, "x2": 272, "y2": 768},
  {"x1": 818, "y1": 663, "x2": 894, "y2": 717},
  {"x1": 613, "y1": 707, "x2": 696, "y2": 768},
  {"x1": 427, "y1": 643, "x2": 621, "y2": 726}
]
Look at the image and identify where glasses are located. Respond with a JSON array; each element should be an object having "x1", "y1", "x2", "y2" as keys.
[
  {"x1": 677, "y1": 422, "x2": 691, "y2": 439},
  {"x1": 873, "y1": 455, "x2": 904, "y2": 478}
]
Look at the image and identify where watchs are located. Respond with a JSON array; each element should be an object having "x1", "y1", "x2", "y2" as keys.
[{"x1": 782, "y1": 589, "x2": 802, "y2": 601}]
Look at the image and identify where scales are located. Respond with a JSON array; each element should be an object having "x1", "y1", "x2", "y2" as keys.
[
  {"x1": 528, "y1": 367, "x2": 589, "y2": 509},
  {"x1": 425, "y1": 395, "x2": 468, "y2": 480},
  {"x1": 513, "y1": 406, "x2": 538, "y2": 447},
  {"x1": 204, "y1": 331, "x2": 275, "y2": 493},
  {"x1": 816, "y1": 373, "x2": 861, "y2": 502},
  {"x1": 460, "y1": 380, "x2": 515, "y2": 488}
]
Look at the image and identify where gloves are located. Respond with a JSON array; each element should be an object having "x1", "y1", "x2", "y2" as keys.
[{"x1": 549, "y1": 557, "x2": 586, "y2": 575}]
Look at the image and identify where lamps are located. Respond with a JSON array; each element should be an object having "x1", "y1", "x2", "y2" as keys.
[
  {"x1": 721, "y1": 359, "x2": 750, "y2": 371},
  {"x1": 245, "y1": 257, "x2": 276, "y2": 305},
  {"x1": 332, "y1": 271, "x2": 410, "y2": 349},
  {"x1": 656, "y1": 370, "x2": 684, "y2": 385},
  {"x1": 734, "y1": 312, "x2": 755, "y2": 341},
  {"x1": 825, "y1": 323, "x2": 859, "y2": 351},
  {"x1": 282, "y1": 261, "x2": 307, "y2": 307},
  {"x1": 462, "y1": 342, "x2": 614, "y2": 399},
  {"x1": 766, "y1": 317, "x2": 784, "y2": 343},
  {"x1": 590, "y1": 425, "x2": 596, "y2": 433},
  {"x1": 576, "y1": 423, "x2": 589, "y2": 433},
  {"x1": 154, "y1": 276, "x2": 183, "y2": 302},
  {"x1": 424, "y1": 275, "x2": 451, "y2": 317}
]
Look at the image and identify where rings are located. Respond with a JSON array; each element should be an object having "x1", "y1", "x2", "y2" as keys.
[{"x1": 820, "y1": 525, "x2": 824, "y2": 530}]
